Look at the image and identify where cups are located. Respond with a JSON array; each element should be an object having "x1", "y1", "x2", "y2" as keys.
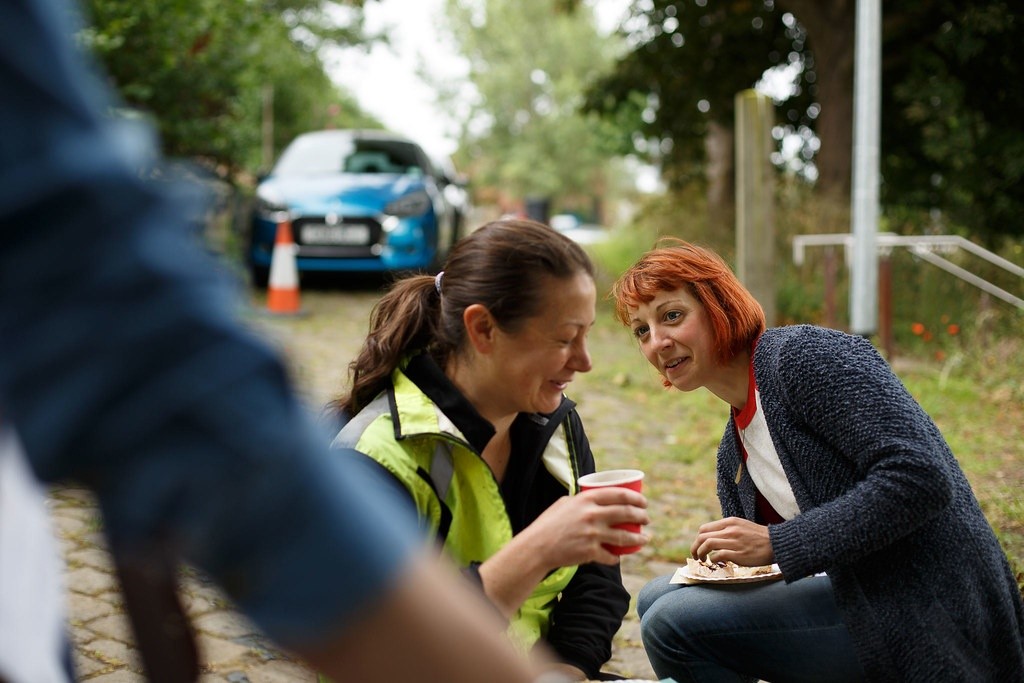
[{"x1": 576, "y1": 469, "x2": 645, "y2": 555}]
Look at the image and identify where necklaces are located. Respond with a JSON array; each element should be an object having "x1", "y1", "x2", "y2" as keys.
[{"x1": 731, "y1": 393, "x2": 749, "y2": 484}]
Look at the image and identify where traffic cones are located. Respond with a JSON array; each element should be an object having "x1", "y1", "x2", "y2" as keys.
[{"x1": 254, "y1": 216, "x2": 315, "y2": 320}]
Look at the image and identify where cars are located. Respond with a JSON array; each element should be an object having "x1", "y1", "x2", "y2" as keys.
[{"x1": 230, "y1": 126, "x2": 467, "y2": 290}]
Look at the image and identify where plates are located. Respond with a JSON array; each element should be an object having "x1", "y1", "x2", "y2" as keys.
[{"x1": 678, "y1": 564, "x2": 782, "y2": 584}]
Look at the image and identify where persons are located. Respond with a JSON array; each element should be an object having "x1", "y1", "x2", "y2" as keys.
[
  {"x1": 2, "y1": 1, "x2": 603, "y2": 682},
  {"x1": 319, "y1": 217, "x2": 645, "y2": 681},
  {"x1": 605, "y1": 235, "x2": 1024, "y2": 678}
]
